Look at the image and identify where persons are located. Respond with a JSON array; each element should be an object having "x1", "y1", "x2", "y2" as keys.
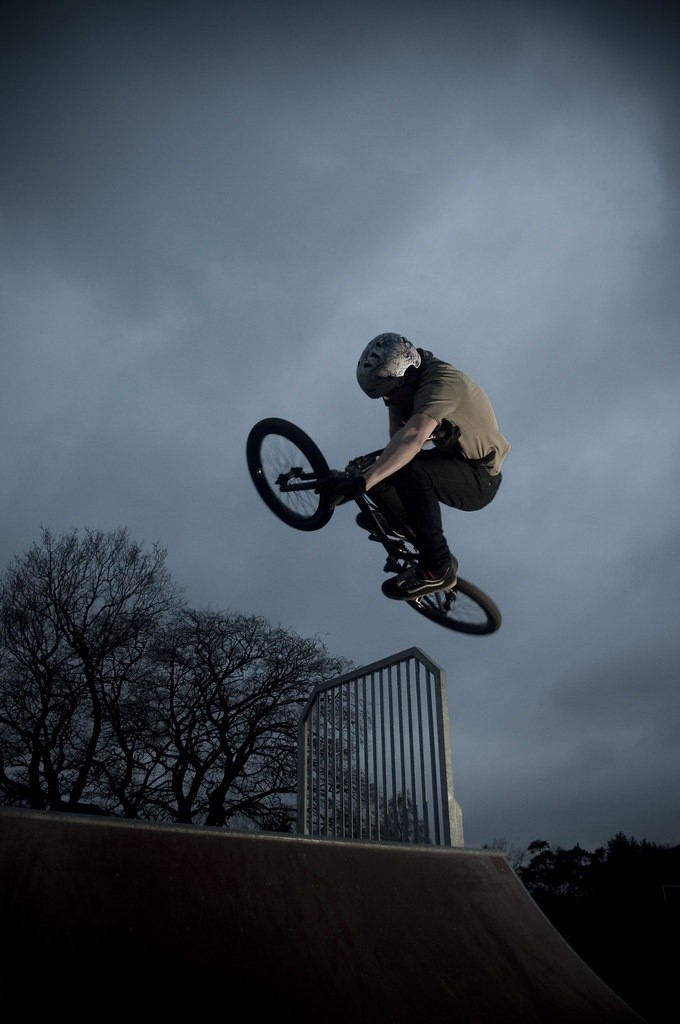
[{"x1": 315, "y1": 333, "x2": 510, "y2": 601}]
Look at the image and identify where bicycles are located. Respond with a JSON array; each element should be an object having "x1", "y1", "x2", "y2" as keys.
[{"x1": 245, "y1": 413, "x2": 503, "y2": 635}]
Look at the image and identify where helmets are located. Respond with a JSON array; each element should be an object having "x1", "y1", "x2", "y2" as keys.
[{"x1": 354, "y1": 333, "x2": 422, "y2": 398}]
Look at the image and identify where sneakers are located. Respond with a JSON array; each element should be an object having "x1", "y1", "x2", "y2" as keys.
[
  {"x1": 449, "y1": 552, "x2": 457, "y2": 569},
  {"x1": 381, "y1": 563, "x2": 456, "y2": 600}
]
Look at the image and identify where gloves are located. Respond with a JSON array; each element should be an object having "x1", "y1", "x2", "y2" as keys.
[{"x1": 312, "y1": 475, "x2": 366, "y2": 509}]
